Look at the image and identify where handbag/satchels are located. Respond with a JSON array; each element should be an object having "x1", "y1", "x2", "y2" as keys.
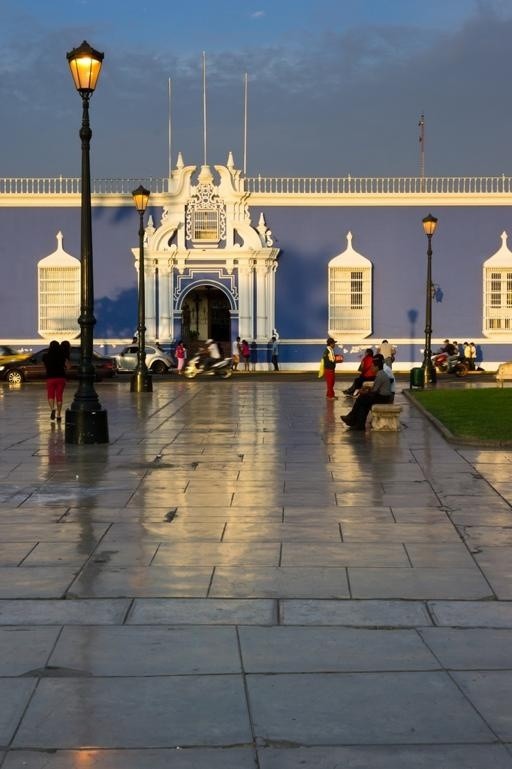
[{"x1": 318, "y1": 359, "x2": 325, "y2": 378}]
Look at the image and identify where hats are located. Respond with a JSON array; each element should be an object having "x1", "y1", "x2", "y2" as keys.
[
  {"x1": 205, "y1": 338, "x2": 213, "y2": 344},
  {"x1": 327, "y1": 338, "x2": 337, "y2": 344}
]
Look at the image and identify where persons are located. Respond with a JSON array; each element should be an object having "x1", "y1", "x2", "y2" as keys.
[
  {"x1": 271, "y1": 337, "x2": 279, "y2": 371},
  {"x1": 341, "y1": 349, "x2": 375, "y2": 399},
  {"x1": 232, "y1": 336, "x2": 241, "y2": 370},
  {"x1": 340, "y1": 354, "x2": 397, "y2": 426},
  {"x1": 241, "y1": 340, "x2": 251, "y2": 371},
  {"x1": 156, "y1": 342, "x2": 161, "y2": 350},
  {"x1": 60, "y1": 341, "x2": 72, "y2": 358},
  {"x1": 132, "y1": 337, "x2": 138, "y2": 344},
  {"x1": 198, "y1": 339, "x2": 222, "y2": 369},
  {"x1": 441, "y1": 339, "x2": 476, "y2": 370},
  {"x1": 43, "y1": 340, "x2": 68, "y2": 422},
  {"x1": 322, "y1": 338, "x2": 344, "y2": 399},
  {"x1": 347, "y1": 359, "x2": 392, "y2": 431},
  {"x1": 378, "y1": 339, "x2": 394, "y2": 370},
  {"x1": 176, "y1": 340, "x2": 187, "y2": 374}
]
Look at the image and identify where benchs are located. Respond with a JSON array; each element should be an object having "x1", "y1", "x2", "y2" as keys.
[
  {"x1": 369, "y1": 402, "x2": 404, "y2": 434},
  {"x1": 362, "y1": 381, "x2": 375, "y2": 390},
  {"x1": 496, "y1": 362, "x2": 512, "y2": 389}
]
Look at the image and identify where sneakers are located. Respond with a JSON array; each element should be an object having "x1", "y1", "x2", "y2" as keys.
[
  {"x1": 326, "y1": 396, "x2": 338, "y2": 401},
  {"x1": 57, "y1": 417, "x2": 61, "y2": 420},
  {"x1": 342, "y1": 391, "x2": 353, "y2": 399},
  {"x1": 51, "y1": 409, "x2": 56, "y2": 420},
  {"x1": 340, "y1": 416, "x2": 366, "y2": 431}
]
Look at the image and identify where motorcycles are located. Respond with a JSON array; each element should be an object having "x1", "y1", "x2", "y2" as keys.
[
  {"x1": 421, "y1": 348, "x2": 469, "y2": 380},
  {"x1": 183, "y1": 346, "x2": 237, "y2": 381},
  {"x1": 126, "y1": 183, "x2": 157, "y2": 392},
  {"x1": 62, "y1": 40, "x2": 111, "y2": 448},
  {"x1": 420, "y1": 212, "x2": 439, "y2": 390}
]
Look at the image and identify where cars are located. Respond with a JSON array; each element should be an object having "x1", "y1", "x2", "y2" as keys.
[
  {"x1": 1, "y1": 343, "x2": 31, "y2": 366},
  {"x1": 2, "y1": 348, "x2": 120, "y2": 385},
  {"x1": 105, "y1": 345, "x2": 174, "y2": 374}
]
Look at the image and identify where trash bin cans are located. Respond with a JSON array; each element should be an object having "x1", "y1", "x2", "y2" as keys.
[{"x1": 410, "y1": 368, "x2": 424, "y2": 389}]
